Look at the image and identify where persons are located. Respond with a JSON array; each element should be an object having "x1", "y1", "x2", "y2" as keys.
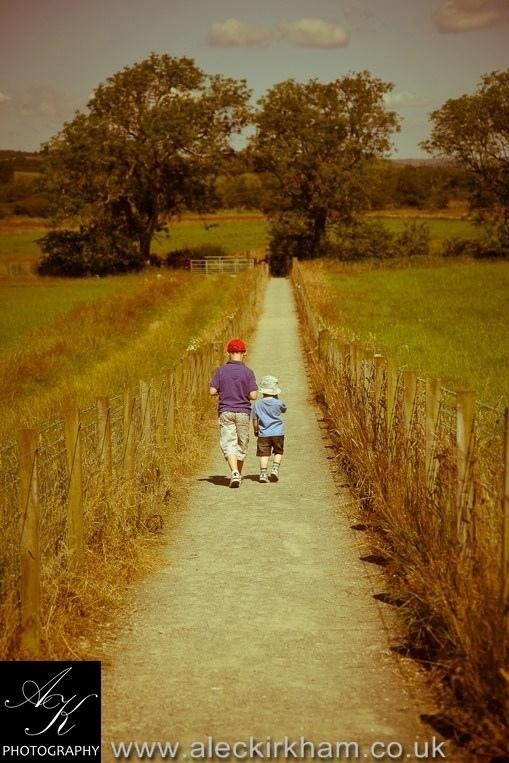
[
  {"x1": 209, "y1": 338, "x2": 258, "y2": 489},
  {"x1": 252, "y1": 375, "x2": 287, "y2": 483}
]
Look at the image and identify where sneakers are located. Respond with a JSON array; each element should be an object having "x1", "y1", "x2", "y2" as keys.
[
  {"x1": 230, "y1": 473, "x2": 241, "y2": 487},
  {"x1": 270, "y1": 468, "x2": 279, "y2": 481},
  {"x1": 259, "y1": 473, "x2": 268, "y2": 482}
]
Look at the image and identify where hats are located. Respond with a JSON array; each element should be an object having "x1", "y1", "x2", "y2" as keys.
[
  {"x1": 227, "y1": 340, "x2": 245, "y2": 352},
  {"x1": 257, "y1": 376, "x2": 281, "y2": 395}
]
